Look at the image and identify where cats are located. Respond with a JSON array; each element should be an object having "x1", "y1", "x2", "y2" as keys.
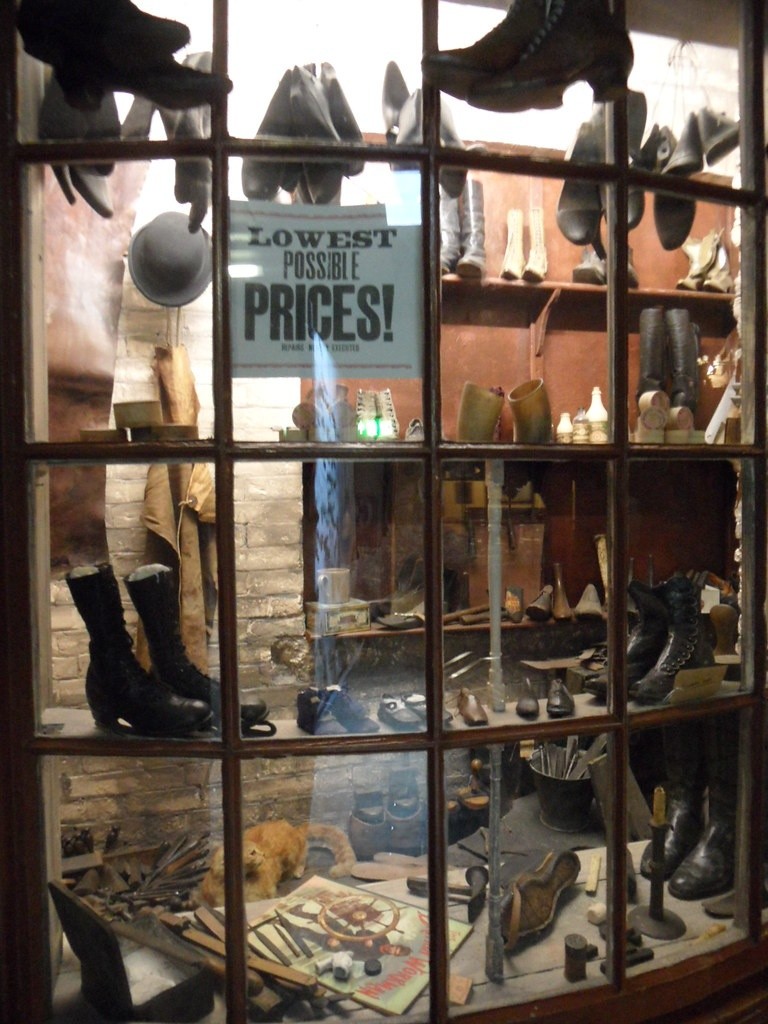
[{"x1": 200, "y1": 819, "x2": 358, "y2": 906}]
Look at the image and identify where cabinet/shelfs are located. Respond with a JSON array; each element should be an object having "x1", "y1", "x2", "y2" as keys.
[
  {"x1": 0, "y1": 0, "x2": 768, "y2": 1024},
  {"x1": 302, "y1": 132, "x2": 740, "y2": 702}
]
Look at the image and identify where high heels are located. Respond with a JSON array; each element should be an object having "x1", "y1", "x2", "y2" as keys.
[{"x1": 36, "y1": 77, "x2": 122, "y2": 218}]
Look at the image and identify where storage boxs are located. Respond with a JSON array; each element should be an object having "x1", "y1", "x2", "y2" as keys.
[{"x1": 47, "y1": 878, "x2": 215, "y2": 1024}]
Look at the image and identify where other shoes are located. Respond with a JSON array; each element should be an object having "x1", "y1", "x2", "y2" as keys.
[
  {"x1": 241, "y1": 62, "x2": 367, "y2": 205},
  {"x1": 421, "y1": 1, "x2": 634, "y2": 113},
  {"x1": 573, "y1": 581, "x2": 605, "y2": 619},
  {"x1": 376, "y1": 693, "x2": 423, "y2": 729},
  {"x1": 526, "y1": 584, "x2": 554, "y2": 623},
  {"x1": 296, "y1": 686, "x2": 348, "y2": 737},
  {"x1": 287, "y1": 904, "x2": 306, "y2": 914},
  {"x1": 381, "y1": 60, "x2": 467, "y2": 199},
  {"x1": 653, "y1": 111, "x2": 704, "y2": 252},
  {"x1": 400, "y1": 691, "x2": 454, "y2": 725},
  {"x1": 697, "y1": 106, "x2": 739, "y2": 166},
  {"x1": 316, "y1": 684, "x2": 380, "y2": 733},
  {"x1": 499, "y1": 847, "x2": 581, "y2": 941}
]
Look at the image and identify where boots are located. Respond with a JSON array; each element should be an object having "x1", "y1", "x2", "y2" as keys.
[
  {"x1": 556, "y1": 99, "x2": 647, "y2": 246},
  {"x1": 678, "y1": 228, "x2": 722, "y2": 289},
  {"x1": 456, "y1": 180, "x2": 487, "y2": 277},
  {"x1": 521, "y1": 208, "x2": 548, "y2": 284},
  {"x1": 551, "y1": 560, "x2": 572, "y2": 620},
  {"x1": 64, "y1": 562, "x2": 214, "y2": 735},
  {"x1": 572, "y1": 244, "x2": 606, "y2": 286},
  {"x1": 122, "y1": 563, "x2": 268, "y2": 732},
  {"x1": 635, "y1": 308, "x2": 668, "y2": 405},
  {"x1": 439, "y1": 186, "x2": 457, "y2": 276},
  {"x1": 501, "y1": 209, "x2": 526, "y2": 280},
  {"x1": 703, "y1": 239, "x2": 731, "y2": 294},
  {"x1": 46, "y1": 29, "x2": 235, "y2": 108},
  {"x1": 583, "y1": 579, "x2": 669, "y2": 696},
  {"x1": 17, "y1": 0, "x2": 191, "y2": 64},
  {"x1": 626, "y1": 249, "x2": 639, "y2": 286},
  {"x1": 628, "y1": 572, "x2": 716, "y2": 706},
  {"x1": 663, "y1": 308, "x2": 702, "y2": 417},
  {"x1": 605, "y1": 123, "x2": 660, "y2": 232}
]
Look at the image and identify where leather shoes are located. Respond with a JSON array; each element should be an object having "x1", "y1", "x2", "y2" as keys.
[
  {"x1": 667, "y1": 819, "x2": 738, "y2": 901},
  {"x1": 640, "y1": 798, "x2": 698, "y2": 884},
  {"x1": 456, "y1": 687, "x2": 488, "y2": 726},
  {"x1": 515, "y1": 675, "x2": 539, "y2": 720},
  {"x1": 546, "y1": 678, "x2": 573, "y2": 717}
]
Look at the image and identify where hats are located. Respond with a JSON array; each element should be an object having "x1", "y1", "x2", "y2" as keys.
[{"x1": 127, "y1": 210, "x2": 213, "y2": 308}]
